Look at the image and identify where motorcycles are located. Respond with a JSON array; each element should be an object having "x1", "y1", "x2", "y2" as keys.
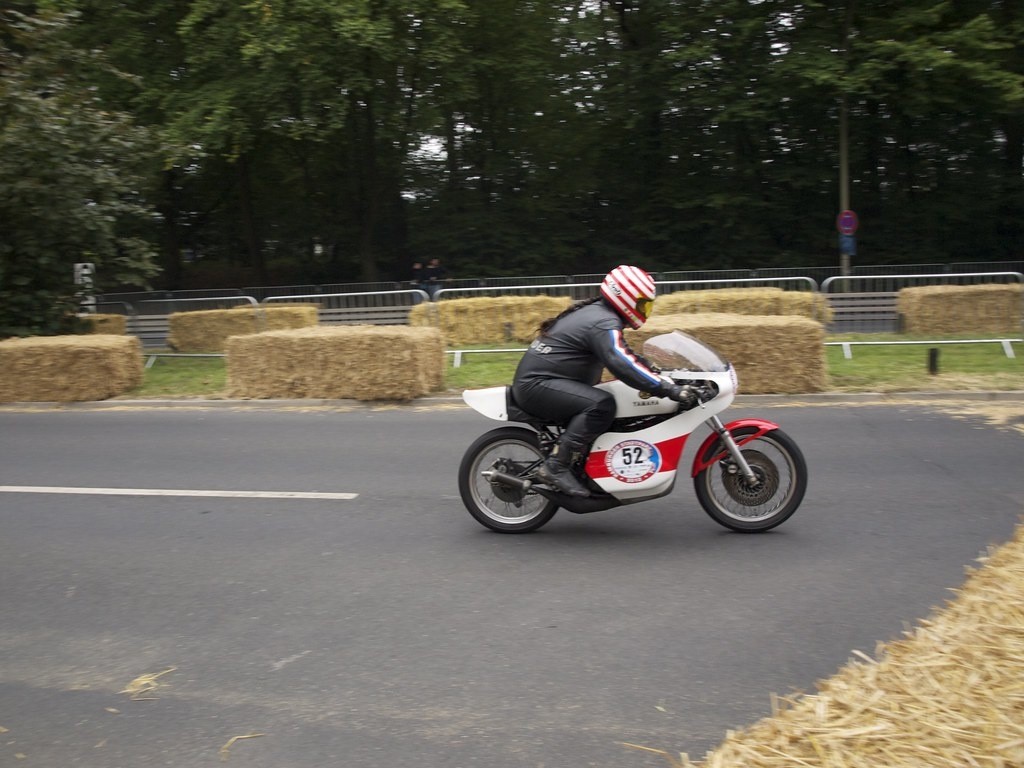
[{"x1": 457, "y1": 327, "x2": 809, "y2": 535}]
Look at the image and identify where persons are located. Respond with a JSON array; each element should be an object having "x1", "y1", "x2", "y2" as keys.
[
  {"x1": 511, "y1": 264, "x2": 705, "y2": 498},
  {"x1": 412, "y1": 258, "x2": 453, "y2": 305}
]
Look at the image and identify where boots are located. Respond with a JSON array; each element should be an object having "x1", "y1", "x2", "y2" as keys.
[{"x1": 535, "y1": 434, "x2": 591, "y2": 498}]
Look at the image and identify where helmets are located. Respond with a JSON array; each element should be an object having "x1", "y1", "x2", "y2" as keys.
[{"x1": 599, "y1": 264, "x2": 656, "y2": 330}]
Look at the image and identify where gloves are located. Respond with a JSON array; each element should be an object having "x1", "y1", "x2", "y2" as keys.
[{"x1": 655, "y1": 379, "x2": 700, "y2": 404}]
